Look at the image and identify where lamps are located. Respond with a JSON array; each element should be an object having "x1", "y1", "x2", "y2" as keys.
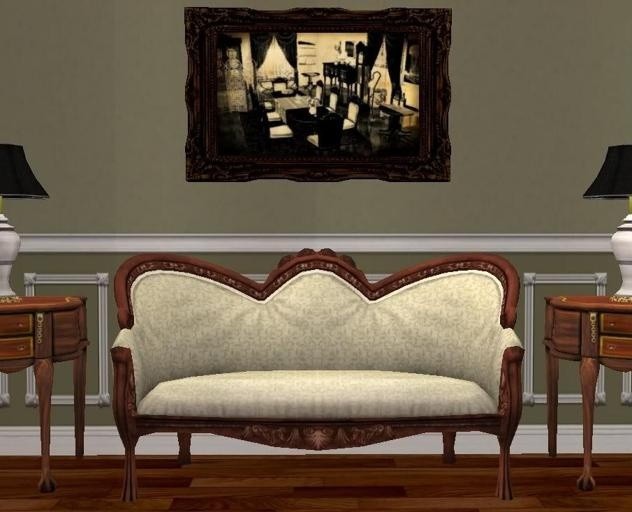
[
  {"x1": 581, "y1": 145, "x2": 631, "y2": 302},
  {"x1": 0, "y1": 142, "x2": 50, "y2": 297}
]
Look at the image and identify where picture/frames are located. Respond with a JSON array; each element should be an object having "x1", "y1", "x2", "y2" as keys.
[{"x1": 181, "y1": 7, "x2": 453, "y2": 181}]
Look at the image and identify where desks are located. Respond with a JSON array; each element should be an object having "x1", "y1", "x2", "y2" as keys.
[
  {"x1": 321, "y1": 60, "x2": 356, "y2": 98},
  {"x1": 377, "y1": 102, "x2": 415, "y2": 139},
  {"x1": 544, "y1": 293, "x2": 632, "y2": 492},
  {"x1": 0, "y1": 296, "x2": 89, "y2": 495}
]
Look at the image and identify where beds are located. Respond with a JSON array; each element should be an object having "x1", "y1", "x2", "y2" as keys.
[{"x1": 301, "y1": 71, "x2": 319, "y2": 90}]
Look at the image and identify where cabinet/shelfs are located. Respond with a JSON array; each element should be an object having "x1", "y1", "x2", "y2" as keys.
[{"x1": 297, "y1": 43, "x2": 318, "y2": 67}]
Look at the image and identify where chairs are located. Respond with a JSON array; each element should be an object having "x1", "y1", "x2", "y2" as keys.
[{"x1": 247, "y1": 78, "x2": 370, "y2": 158}]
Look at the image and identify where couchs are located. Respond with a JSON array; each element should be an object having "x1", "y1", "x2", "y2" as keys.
[{"x1": 106, "y1": 249, "x2": 525, "y2": 507}]
